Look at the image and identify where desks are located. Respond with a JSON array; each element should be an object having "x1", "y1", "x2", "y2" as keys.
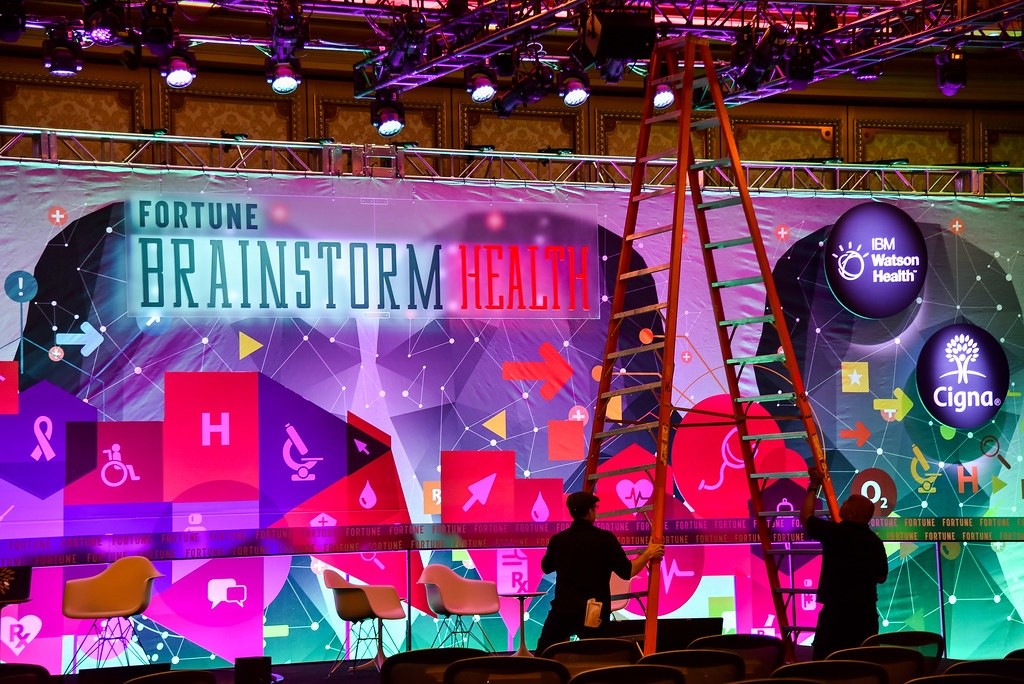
[{"x1": 496, "y1": 592, "x2": 548, "y2": 656}]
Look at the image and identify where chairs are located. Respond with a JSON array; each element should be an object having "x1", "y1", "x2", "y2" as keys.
[
  {"x1": 416, "y1": 564, "x2": 501, "y2": 656},
  {"x1": 323, "y1": 569, "x2": 406, "y2": 676},
  {"x1": 379, "y1": 632, "x2": 1024, "y2": 684},
  {"x1": 61, "y1": 556, "x2": 166, "y2": 676}
]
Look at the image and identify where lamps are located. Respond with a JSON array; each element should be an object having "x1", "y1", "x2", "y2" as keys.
[
  {"x1": 86, "y1": 0, "x2": 129, "y2": 47},
  {"x1": 271, "y1": 1, "x2": 311, "y2": 55},
  {"x1": 0, "y1": 0, "x2": 28, "y2": 45},
  {"x1": 381, "y1": 13, "x2": 426, "y2": 77},
  {"x1": 557, "y1": 62, "x2": 591, "y2": 107},
  {"x1": 786, "y1": 53, "x2": 813, "y2": 91},
  {"x1": 40, "y1": 27, "x2": 82, "y2": 75},
  {"x1": 735, "y1": 23, "x2": 792, "y2": 93},
  {"x1": 141, "y1": 1, "x2": 181, "y2": 54},
  {"x1": 641, "y1": 73, "x2": 675, "y2": 109},
  {"x1": 370, "y1": 88, "x2": 406, "y2": 137},
  {"x1": 814, "y1": 4, "x2": 837, "y2": 34},
  {"x1": 493, "y1": 67, "x2": 554, "y2": 117},
  {"x1": 119, "y1": 48, "x2": 143, "y2": 70},
  {"x1": 462, "y1": 65, "x2": 498, "y2": 104},
  {"x1": 854, "y1": 63, "x2": 882, "y2": 80},
  {"x1": 935, "y1": 57, "x2": 968, "y2": 99},
  {"x1": 570, "y1": 13, "x2": 655, "y2": 87},
  {"x1": 265, "y1": 55, "x2": 301, "y2": 95},
  {"x1": 161, "y1": 38, "x2": 200, "y2": 89}
]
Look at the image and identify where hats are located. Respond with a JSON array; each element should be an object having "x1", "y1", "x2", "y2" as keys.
[{"x1": 566, "y1": 492, "x2": 600, "y2": 510}]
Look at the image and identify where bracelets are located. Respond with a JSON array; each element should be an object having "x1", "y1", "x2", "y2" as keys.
[{"x1": 806, "y1": 484, "x2": 819, "y2": 495}]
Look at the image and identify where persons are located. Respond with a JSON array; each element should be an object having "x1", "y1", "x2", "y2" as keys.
[
  {"x1": 799, "y1": 463, "x2": 889, "y2": 661},
  {"x1": 534, "y1": 492, "x2": 665, "y2": 657}
]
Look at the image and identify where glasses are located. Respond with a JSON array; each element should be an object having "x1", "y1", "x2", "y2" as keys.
[{"x1": 590, "y1": 505, "x2": 600, "y2": 509}]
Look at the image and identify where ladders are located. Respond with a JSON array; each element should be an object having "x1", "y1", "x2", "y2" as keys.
[{"x1": 583, "y1": 37, "x2": 841, "y2": 665}]
